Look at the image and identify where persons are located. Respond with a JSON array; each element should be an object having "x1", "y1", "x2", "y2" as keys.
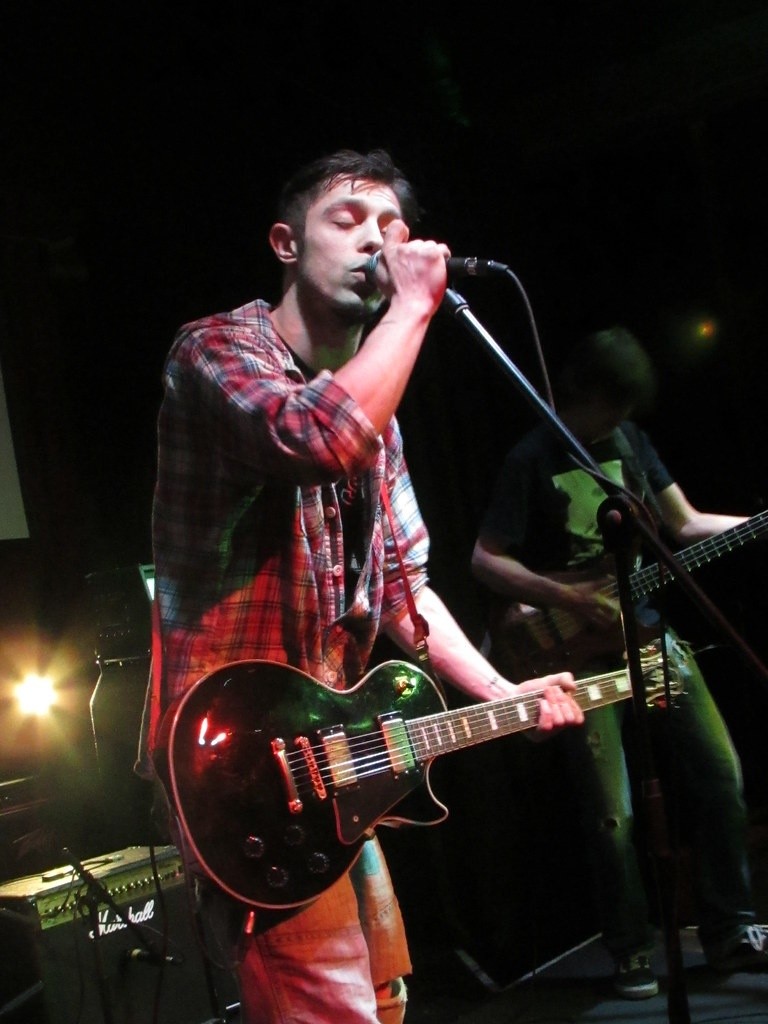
[
  {"x1": 422, "y1": 324, "x2": 767, "y2": 1000},
  {"x1": 132, "y1": 148, "x2": 586, "y2": 1023}
]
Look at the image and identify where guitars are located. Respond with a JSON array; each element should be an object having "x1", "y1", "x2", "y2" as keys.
[
  {"x1": 452, "y1": 507, "x2": 768, "y2": 683},
  {"x1": 151, "y1": 626, "x2": 700, "y2": 918}
]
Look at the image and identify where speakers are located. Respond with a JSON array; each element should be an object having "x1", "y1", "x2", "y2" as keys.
[{"x1": 0, "y1": 842, "x2": 244, "y2": 1024}]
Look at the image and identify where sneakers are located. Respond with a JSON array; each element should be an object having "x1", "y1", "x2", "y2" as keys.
[
  {"x1": 615, "y1": 947, "x2": 660, "y2": 997},
  {"x1": 722, "y1": 924, "x2": 768, "y2": 966}
]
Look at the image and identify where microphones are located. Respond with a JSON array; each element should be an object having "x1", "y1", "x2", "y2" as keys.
[
  {"x1": 365, "y1": 248, "x2": 510, "y2": 286},
  {"x1": 122, "y1": 947, "x2": 181, "y2": 966}
]
